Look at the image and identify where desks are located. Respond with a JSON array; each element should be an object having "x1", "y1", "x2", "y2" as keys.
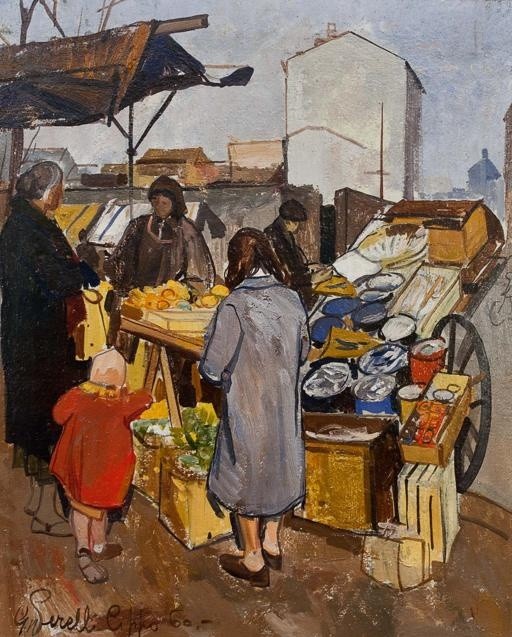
[{"x1": 119, "y1": 290, "x2": 224, "y2": 424}]
